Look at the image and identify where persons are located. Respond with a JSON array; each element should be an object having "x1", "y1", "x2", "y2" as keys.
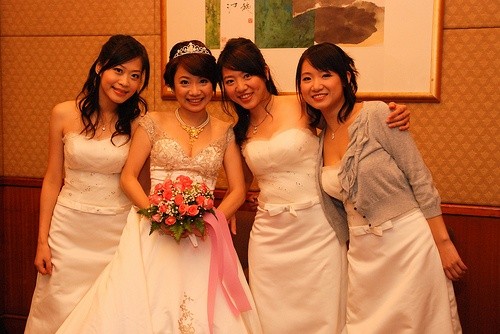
[
  {"x1": 55, "y1": 39, "x2": 266, "y2": 334},
  {"x1": 23, "y1": 34, "x2": 152, "y2": 334},
  {"x1": 215, "y1": 36, "x2": 412, "y2": 334},
  {"x1": 294, "y1": 41, "x2": 471, "y2": 334}
]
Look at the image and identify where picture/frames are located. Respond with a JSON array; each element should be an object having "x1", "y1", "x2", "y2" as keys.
[{"x1": 160, "y1": 0, "x2": 446, "y2": 103}]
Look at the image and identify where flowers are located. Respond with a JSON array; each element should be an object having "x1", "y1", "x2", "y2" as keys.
[{"x1": 136, "y1": 174, "x2": 219, "y2": 242}]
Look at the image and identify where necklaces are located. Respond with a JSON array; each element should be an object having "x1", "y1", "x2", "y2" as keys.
[
  {"x1": 248, "y1": 94, "x2": 274, "y2": 135},
  {"x1": 174, "y1": 107, "x2": 210, "y2": 139},
  {"x1": 99, "y1": 112, "x2": 117, "y2": 131},
  {"x1": 326, "y1": 120, "x2": 342, "y2": 140}
]
